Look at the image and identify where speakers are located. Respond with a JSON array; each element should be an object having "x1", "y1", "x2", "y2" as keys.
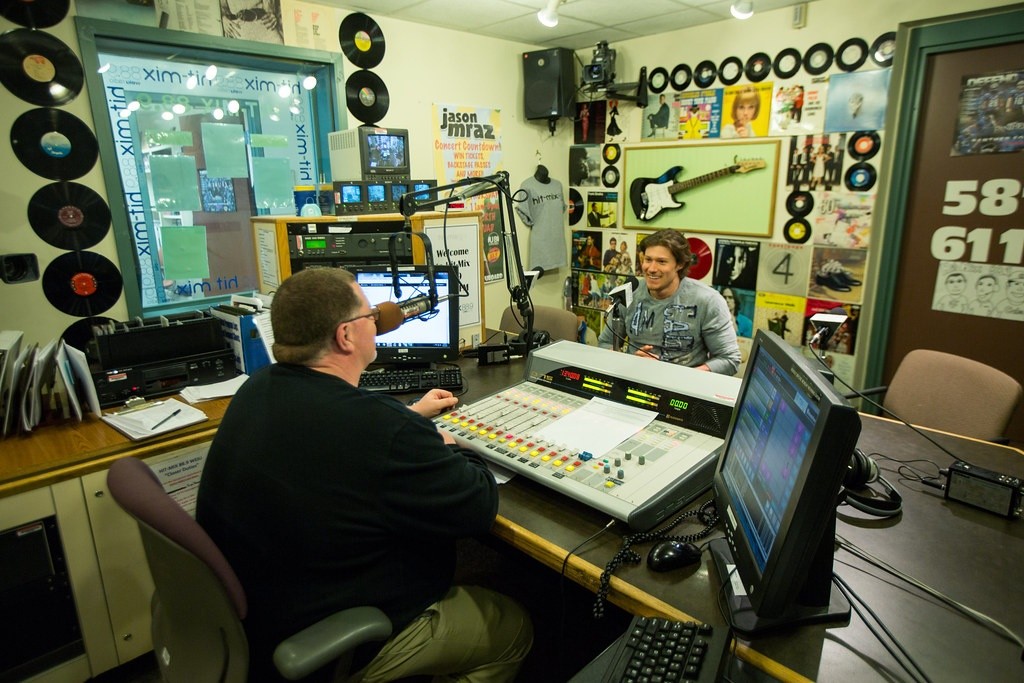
[{"x1": 523, "y1": 47, "x2": 577, "y2": 120}]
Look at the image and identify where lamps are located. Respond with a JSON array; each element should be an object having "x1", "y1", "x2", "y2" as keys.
[
  {"x1": 729, "y1": 0, "x2": 754, "y2": 20},
  {"x1": 537, "y1": 0, "x2": 563, "y2": 27}
]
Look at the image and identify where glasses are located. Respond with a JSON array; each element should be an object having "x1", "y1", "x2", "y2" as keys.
[{"x1": 332, "y1": 305, "x2": 382, "y2": 342}]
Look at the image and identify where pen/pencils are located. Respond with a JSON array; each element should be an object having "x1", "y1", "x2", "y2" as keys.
[{"x1": 151, "y1": 409, "x2": 181, "y2": 430}]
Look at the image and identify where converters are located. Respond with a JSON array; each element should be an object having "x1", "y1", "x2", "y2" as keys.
[
  {"x1": 944, "y1": 460, "x2": 1024, "y2": 520},
  {"x1": 477, "y1": 345, "x2": 510, "y2": 366}
]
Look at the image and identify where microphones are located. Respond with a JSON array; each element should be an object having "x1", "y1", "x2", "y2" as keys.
[
  {"x1": 375, "y1": 293, "x2": 457, "y2": 336},
  {"x1": 809, "y1": 307, "x2": 849, "y2": 345},
  {"x1": 524, "y1": 266, "x2": 545, "y2": 290},
  {"x1": 604, "y1": 277, "x2": 639, "y2": 318}
]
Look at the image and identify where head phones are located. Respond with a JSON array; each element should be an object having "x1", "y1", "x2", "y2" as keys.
[
  {"x1": 839, "y1": 448, "x2": 903, "y2": 516},
  {"x1": 508, "y1": 328, "x2": 551, "y2": 355}
]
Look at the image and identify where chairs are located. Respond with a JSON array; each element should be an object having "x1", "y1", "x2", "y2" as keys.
[
  {"x1": 500, "y1": 305, "x2": 584, "y2": 345},
  {"x1": 108, "y1": 458, "x2": 392, "y2": 683},
  {"x1": 842, "y1": 348, "x2": 1023, "y2": 444}
]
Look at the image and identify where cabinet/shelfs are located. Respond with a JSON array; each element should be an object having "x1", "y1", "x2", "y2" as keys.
[{"x1": 0, "y1": 380, "x2": 236, "y2": 683}]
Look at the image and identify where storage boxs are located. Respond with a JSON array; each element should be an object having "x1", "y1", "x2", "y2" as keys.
[{"x1": 209, "y1": 307, "x2": 272, "y2": 377}]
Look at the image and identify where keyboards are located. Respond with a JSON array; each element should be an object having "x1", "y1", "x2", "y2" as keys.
[
  {"x1": 357, "y1": 368, "x2": 463, "y2": 393},
  {"x1": 601, "y1": 615, "x2": 735, "y2": 683}
]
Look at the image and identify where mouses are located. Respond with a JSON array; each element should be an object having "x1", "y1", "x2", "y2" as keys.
[
  {"x1": 646, "y1": 540, "x2": 703, "y2": 573},
  {"x1": 407, "y1": 397, "x2": 422, "y2": 406}
]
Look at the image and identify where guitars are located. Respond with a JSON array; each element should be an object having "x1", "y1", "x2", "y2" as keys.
[{"x1": 629, "y1": 158, "x2": 766, "y2": 222}]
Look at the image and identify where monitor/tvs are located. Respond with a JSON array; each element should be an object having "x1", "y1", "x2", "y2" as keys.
[
  {"x1": 709, "y1": 328, "x2": 862, "y2": 636},
  {"x1": 328, "y1": 126, "x2": 438, "y2": 203},
  {"x1": 346, "y1": 266, "x2": 458, "y2": 370}
]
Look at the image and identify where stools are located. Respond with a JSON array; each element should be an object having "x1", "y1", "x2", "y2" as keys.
[{"x1": 653, "y1": 126, "x2": 667, "y2": 138}]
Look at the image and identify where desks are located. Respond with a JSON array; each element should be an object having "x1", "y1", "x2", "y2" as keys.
[{"x1": 383, "y1": 324, "x2": 1024, "y2": 683}]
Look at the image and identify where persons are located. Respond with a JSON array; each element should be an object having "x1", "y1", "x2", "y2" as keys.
[
  {"x1": 514, "y1": 165, "x2": 571, "y2": 273},
  {"x1": 647, "y1": 94, "x2": 670, "y2": 138},
  {"x1": 717, "y1": 244, "x2": 748, "y2": 287},
  {"x1": 721, "y1": 87, "x2": 760, "y2": 137},
  {"x1": 579, "y1": 235, "x2": 601, "y2": 268},
  {"x1": 603, "y1": 238, "x2": 633, "y2": 274},
  {"x1": 587, "y1": 202, "x2": 614, "y2": 227},
  {"x1": 195, "y1": 268, "x2": 539, "y2": 682},
  {"x1": 789, "y1": 144, "x2": 841, "y2": 186},
  {"x1": 580, "y1": 104, "x2": 590, "y2": 142},
  {"x1": 570, "y1": 147, "x2": 598, "y2": 186},
  {"x1": 805, "y1": 323, "x2": 852, "y2": 355},
  {"x1": 220, "y1": 0, "x2": 284, "y2": 45},
  {"x1": 581, "y1": 273, "x2": 624, "y2": 309},
  {"x1": 597, "y1": 228, "x2": 743, "y2": 376},
  {"x1": 775, "y1": 85, "x2": 805, "y2": 123},
  {"x1": 719, "y1": 287, "x2": 753, "y2": 337},
  {"x1": 950, "y1": 80, "x2": 1024, "y2": 157}
]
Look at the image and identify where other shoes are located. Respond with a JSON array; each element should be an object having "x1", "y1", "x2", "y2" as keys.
[
  {"x1": 648, "y1": 132, "x2": 654, "y2": 137},
  {"x1": 830, "y1": 271, "x2": 862, "y2": 286},
  {"x1": 815, "y1": 274, "x2": 851, "y2": 292}
]
